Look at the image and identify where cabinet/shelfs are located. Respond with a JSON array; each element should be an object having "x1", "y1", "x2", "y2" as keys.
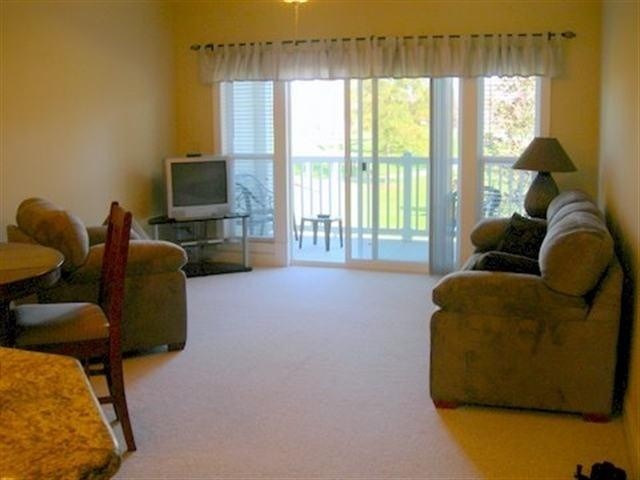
[{"x1": 149, "y1": 212, "x2": 256, "y2": 278}]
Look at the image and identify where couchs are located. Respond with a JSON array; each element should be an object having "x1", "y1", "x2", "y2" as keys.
[{"x1": 429, "y1": 187, "x2": 626, "y2": 424}]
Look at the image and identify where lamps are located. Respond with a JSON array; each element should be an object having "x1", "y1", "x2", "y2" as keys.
[{"x1": 512, "y1": 135, "x2": 577, "y2": 219}]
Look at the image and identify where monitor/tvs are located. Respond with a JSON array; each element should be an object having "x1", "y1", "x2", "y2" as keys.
[{"x1": 166, "y1": 156, "x2": 234, "y2": 221}]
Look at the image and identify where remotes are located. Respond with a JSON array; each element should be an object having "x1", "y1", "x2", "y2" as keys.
[{"x1": 187, "y1": 153, "x2": 202, "y2": 157}]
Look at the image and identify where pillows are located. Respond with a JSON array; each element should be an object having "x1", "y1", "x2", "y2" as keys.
[
  {"x1": 470, "y1": 250, "x2": 539, "y2": 277},
  {"x1": 490, "y1": 210, "x2": 547, "y2": 257}
]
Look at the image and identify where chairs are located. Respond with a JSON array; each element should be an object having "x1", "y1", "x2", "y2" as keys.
[
  {"x1": 234, "y1": 173, "x2": 298, "y2": 244},
  {"x1": 452, "y1": 186, "x2": 502, "y2": 242},
  {"x1": 8, "y1": 196, "x2": 187, "y2": 351},
  {"x1": 10, "y1": 199, "x2": 138, "y2": 452}
]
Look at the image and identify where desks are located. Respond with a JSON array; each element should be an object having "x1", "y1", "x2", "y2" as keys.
[
  {"x1": 0, "y1": 238, "x2": 65, "y2": 302},
  {"x1": 299, "y1": 214, "x2": 344, "y2": 253}
]
